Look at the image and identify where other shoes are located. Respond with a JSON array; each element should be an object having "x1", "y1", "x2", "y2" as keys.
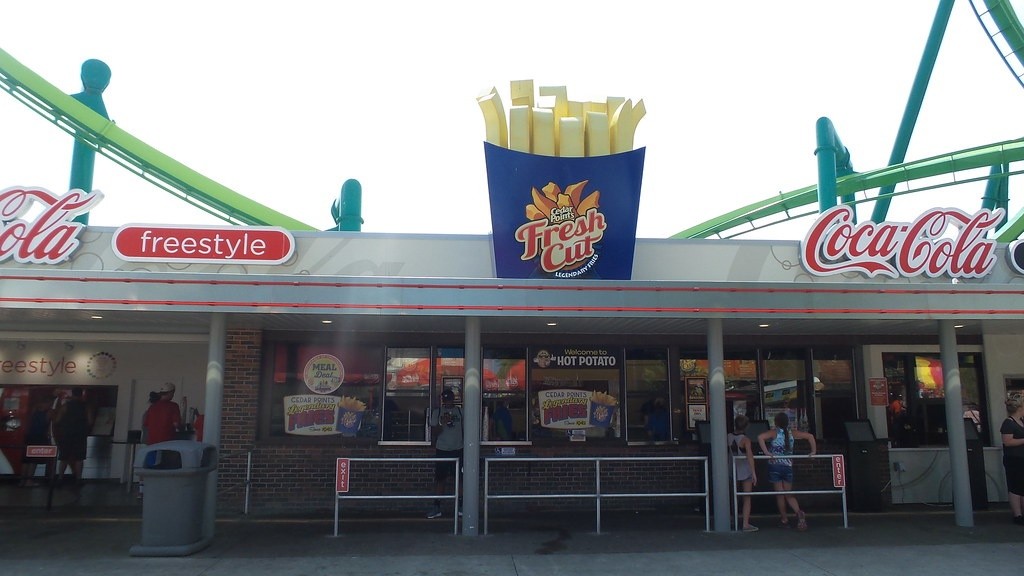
[
  {"x1": 1013, "y1": 515, "x2": 1024, "y2": 525},
  {"x1": 137, "y1": 492, "x2": 143, "y2": 498},
  {"x1": 798, "y1": 510, "x2": 808, "y2": 532},
  {"x1": 776, "y1": 518, "x2": 793, "y2": 525},
  {"x1": 19, "y1": 479, "x2": 40, "y2": 487}
]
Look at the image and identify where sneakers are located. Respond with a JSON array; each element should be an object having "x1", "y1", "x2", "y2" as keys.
[
  {"x1": 743, "y1": 525, "x2": 759, "y2": 532},
  {"x1": 425, "y1": 510, "x2": 442, "y2": 519},
  {"x1": 458, "y1": 511, "x2": 462, "y2": 516}
]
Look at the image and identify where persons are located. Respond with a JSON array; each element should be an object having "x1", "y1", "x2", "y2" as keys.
[
  {"x1": 381, "y1": 397, "x2": 401, "y2": 439},
  {"x1": 424, "y1": 389, "x2": 465, "y2": 519},
  {"x1": 136, "y1": 383, "x2": 182, "y2": 498},
  {"x1": 888, "y1": 395, "x2": 903, "y2": 448},
  {"x1": 52, "y1": 387, "x2": 95, "y2": 480},
  {"x1": 757, "y1": 412, "x2": 816, "y2": 532},
  {"x1": 17, "y1": 413, "x2": 47, "y2": 488},
  {"x1": 644, "y1": 396, "x2": 669, "y2": 441},
  {"x1": 727, "y1": 415, "x2": 759, "y2": 532},
  {"x1": 999, "y1": 391, "x2": 1024, "y2": 526},
  {"x1": 493, "y1": 399, "x2": 513, "y2": 440}
]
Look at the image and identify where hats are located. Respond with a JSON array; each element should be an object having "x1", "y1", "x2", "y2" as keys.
[
  {"x1": 441, "y1": 390, "x2": 454, "y2": 400},
  {"x1": 160, "y1": 382, "x2": 175, "y2": 392}
]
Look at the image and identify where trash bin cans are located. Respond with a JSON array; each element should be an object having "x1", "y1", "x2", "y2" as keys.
[{"x1": 129, "y1": 439, "x2": 220, "y2": 557}]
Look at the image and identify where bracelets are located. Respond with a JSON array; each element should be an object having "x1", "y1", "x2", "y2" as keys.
[{"x1": 439, "y1": 421, "x2": 442, "y2": 425}]
